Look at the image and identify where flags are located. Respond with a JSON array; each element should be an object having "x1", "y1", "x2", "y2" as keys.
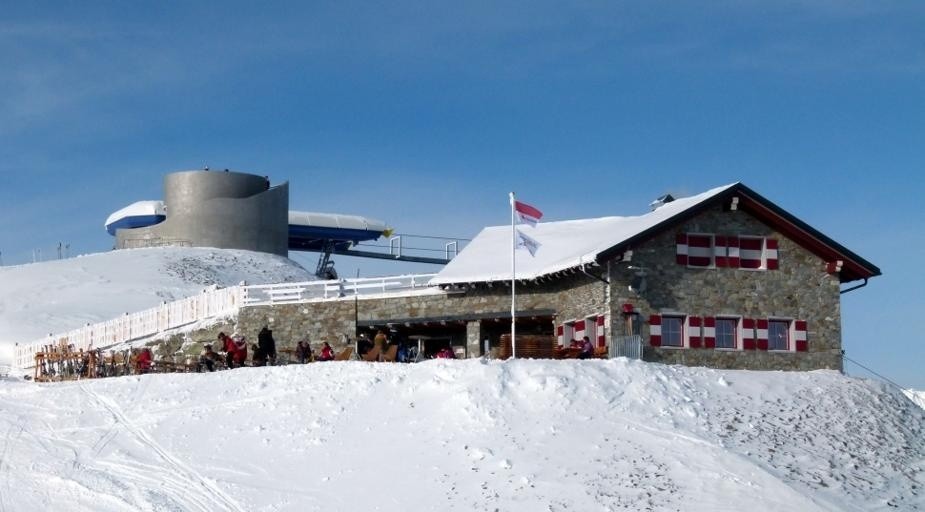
[
  {"x1": 514, "y1": 229, "x2": 542, "y2": 256},
  {"x1": 514, "y1": 201, "x2": 542, "y2": 228}
]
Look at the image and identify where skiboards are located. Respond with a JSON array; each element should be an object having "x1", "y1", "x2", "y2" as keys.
[{"x1": 40, "y1": 332, "x2": 132, "y2": 381}]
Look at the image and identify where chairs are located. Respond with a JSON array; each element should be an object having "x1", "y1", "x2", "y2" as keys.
[
  {"x1": 361, "y1": 345, "x2": 398, "y2": 362},
  {"x1": 87, "y1": 347, "x2": 354, "y2": 379}
]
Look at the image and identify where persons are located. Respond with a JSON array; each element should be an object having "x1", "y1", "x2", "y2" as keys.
[
  {"x1": 432, "y1": 347, "x2": 454, "y2": 358},
  {"x1": 358, "y1": 323, "x2": 409, "y2": 360},
  {"x1": 314, "y1": 342, "x2": 334, "y2": 362},
  {"x1": 295, "y1": 338, "x2": 311, "y2": 363},
  {"x1": 138, "y1": 347, "x2": 154, "y2": 373},
  {"x1": 200, "y1": 325, "x2": 277, "y2": 372},
  {"x1": 576, "y1": 335, "x2": 594, "y2": 360},
  {"x1": 569, "y1": 339, "x2": 583, "y2": 348}
]
